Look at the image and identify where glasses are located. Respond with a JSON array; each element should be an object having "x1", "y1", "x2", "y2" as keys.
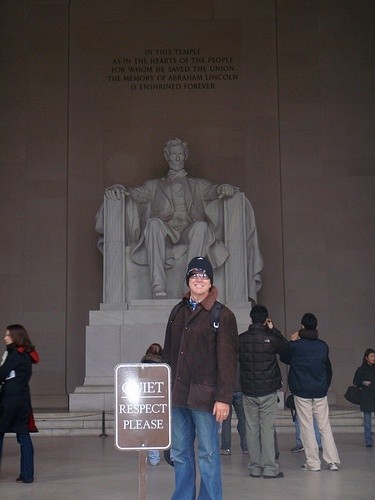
[{"x1": 191, "y1": 272, "x2": 209, "y2": 281}]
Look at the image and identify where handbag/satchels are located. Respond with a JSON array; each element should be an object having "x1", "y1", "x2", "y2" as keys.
[
  {"x1": 286, "y1": 395, "x2": 296, "y2": 409},
  {"x1": 344, "y1": 382, "x2": 365, "y2": 404}
]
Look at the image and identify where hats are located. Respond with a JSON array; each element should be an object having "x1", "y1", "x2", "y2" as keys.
[{"x1": 186, "y1": 257, "x2": 214, "y2": 288}]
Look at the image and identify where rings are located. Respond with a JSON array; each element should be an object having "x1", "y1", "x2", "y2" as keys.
[{"x1": 225, "y1": 412, "x2": 228, "y2": 415}]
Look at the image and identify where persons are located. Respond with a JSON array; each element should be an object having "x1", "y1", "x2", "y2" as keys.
[
  {"x1": 292, "y1": 410, "x2": 323, "y2": 453},
  {"x1": 0, "y1": 324, "x2": 38, "y2": 482},
  {"x1": 219, "y1": 394, "x2": 249, "y2": 455},
  {"x1": 353, "y1": 348, "x2": 375, "y2": 447},
  {"x1": 140, "y1": 343, "x2": 172, "y2": 465},
  {"x1": 102, "y1": 139, "x2": 240, "y2": 300},
  {"x1": 279, "y1": 313, "x2": 342, "y2": 471},
  {"x1": 159, "y1": 256, "x2": 239, "y2": 500},
  {"x1": 239, "y1": 305, "x2": 289, "y2": 478}
]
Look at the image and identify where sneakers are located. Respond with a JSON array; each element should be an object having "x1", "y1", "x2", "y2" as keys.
[
  {"x1": 327, "y1": 463, "x2": 339, "y2": 471},
  {"x1": 301, "y1": 464, "x2": 321, "y2": 471},
  {"x1": 291, "y1": 446, "x2": 304, "y2": 452}
]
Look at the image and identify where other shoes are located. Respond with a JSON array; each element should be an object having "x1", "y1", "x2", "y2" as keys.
[
  {"x1": 164, "y1": 449, "x2": 173, "y2": 466},
  {"x1": 275, "y1": 453, "x2": 280, "y2": 458},
  {"x1": 366, "y1": 444, "x2": 373, "y2": 447},
  {"x1": 151, "y1": 463, "x2": 158, "y2": 465},
  {"x1": 242, "y1": 448, "x2": 249, "y2": 455},
  {"x1": 250, "y1": 473, "x2": 261, "y2": 477},
  {"x1": 220, "y1": 448, "x2": 231, "y2": 455},
  {"x1": 263, "y1": 472, "x2": 284, "y2": 478},
  {"x1": 15, "y1": 477, "x2": 34, "y2": 483}
]
